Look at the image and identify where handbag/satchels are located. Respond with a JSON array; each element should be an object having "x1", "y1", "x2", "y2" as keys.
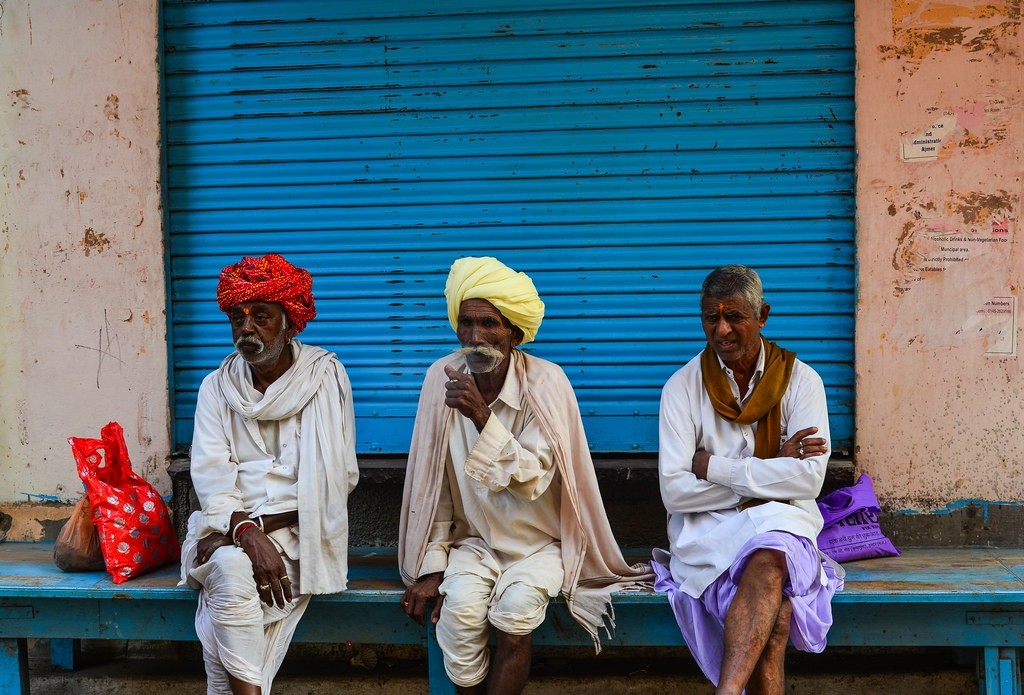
[
  {"x1": 67, "y1": 421, "x2": 181, "y2": 584},
  {"x1": 52, "y1": 494, "x2": 106, "y2": 573},
  {"x1": 816, "y1": 474, "x2": 900, "y2": 563}
]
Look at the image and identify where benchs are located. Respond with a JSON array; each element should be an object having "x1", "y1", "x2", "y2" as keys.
[{"x1": 0, "y1": 552, "x2": 1024, "y2": 695}]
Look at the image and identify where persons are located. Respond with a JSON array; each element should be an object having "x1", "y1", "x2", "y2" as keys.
[
  {"x1": 399, "y1": 255, "x2": 568, "y2": 695},
  {"x1": 658, "y1": 265, "x2": 831, "y2": 695},
  {"x1": 176, "y1": 255, "x2": 359, "y2": 694}
]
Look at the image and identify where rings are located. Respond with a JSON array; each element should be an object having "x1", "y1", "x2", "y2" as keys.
[
  {"x1": 404, "y1": 602, "x2": 408, "y2": 606},
  {"x1": 280, "y1": 576, "x2": 287, "y2": 580},
  {"x1": 799, "y1": 448, "x2": 804, "y2": 455},
  {"x1": 800, "y1": 441, "x2": 804, "y2": 447},
  {"x1": 260, "y1": 584, "x2": 269, "y2": 590}
]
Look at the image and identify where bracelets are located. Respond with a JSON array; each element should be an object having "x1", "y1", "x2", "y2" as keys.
[{"x1": 233, "y1": 517, "x2": 264, "y2": 547}]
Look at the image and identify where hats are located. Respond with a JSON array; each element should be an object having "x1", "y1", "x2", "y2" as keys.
[
  {"x1": 216, "y1": 254, "x2": 316, "y2": 337},
  {"x1": 444, "y1": 256, "x2": 545, "y2": 346}
]
